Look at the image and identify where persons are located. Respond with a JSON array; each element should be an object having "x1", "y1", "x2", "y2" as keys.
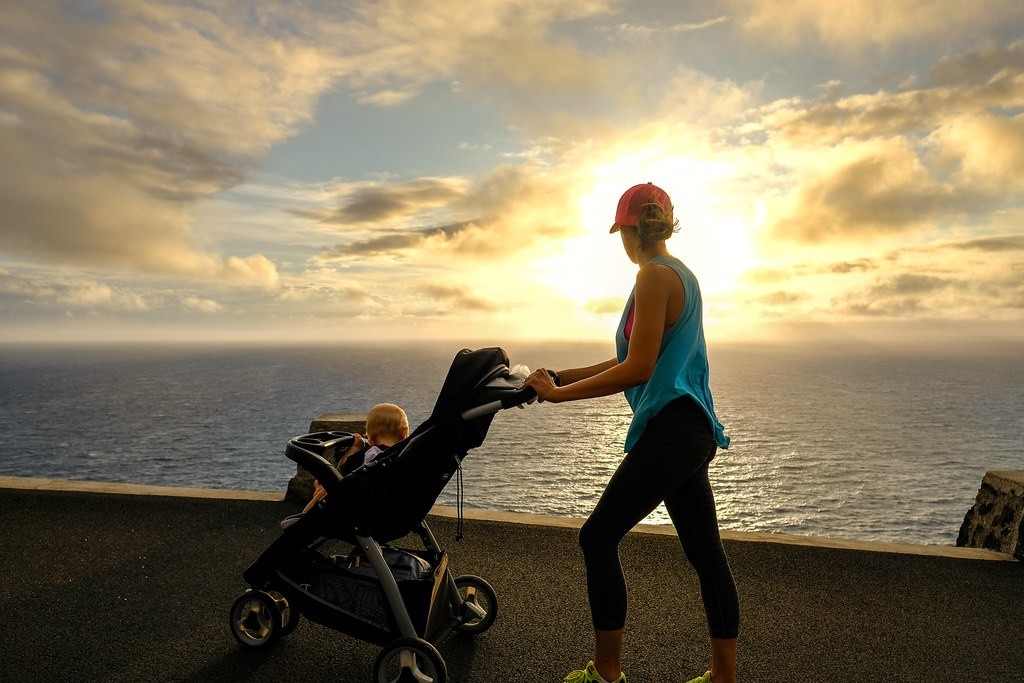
[
  {"x1": 281, "y1": 402, "x2": 409, "y2": 530},
  {"x1": 518, "y1": 182, "x2": 740, "y2": 683}
]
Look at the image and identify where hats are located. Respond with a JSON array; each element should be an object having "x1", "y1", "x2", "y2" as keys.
[{"x1": 609, "y1": 182, "x2": 673, "y2": 234}]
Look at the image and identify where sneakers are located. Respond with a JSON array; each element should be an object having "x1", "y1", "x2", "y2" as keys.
[
  {"x1": 686, "y1": 671, "x2": 712, "y2": 683},
  {"x1": 564, "y1": 661, "x2": 626, "y2": 683}
]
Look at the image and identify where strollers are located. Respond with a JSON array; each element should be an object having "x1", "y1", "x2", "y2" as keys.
[{"x1": 228, "y1": 351, "x2": 558, "y2": 680}]
